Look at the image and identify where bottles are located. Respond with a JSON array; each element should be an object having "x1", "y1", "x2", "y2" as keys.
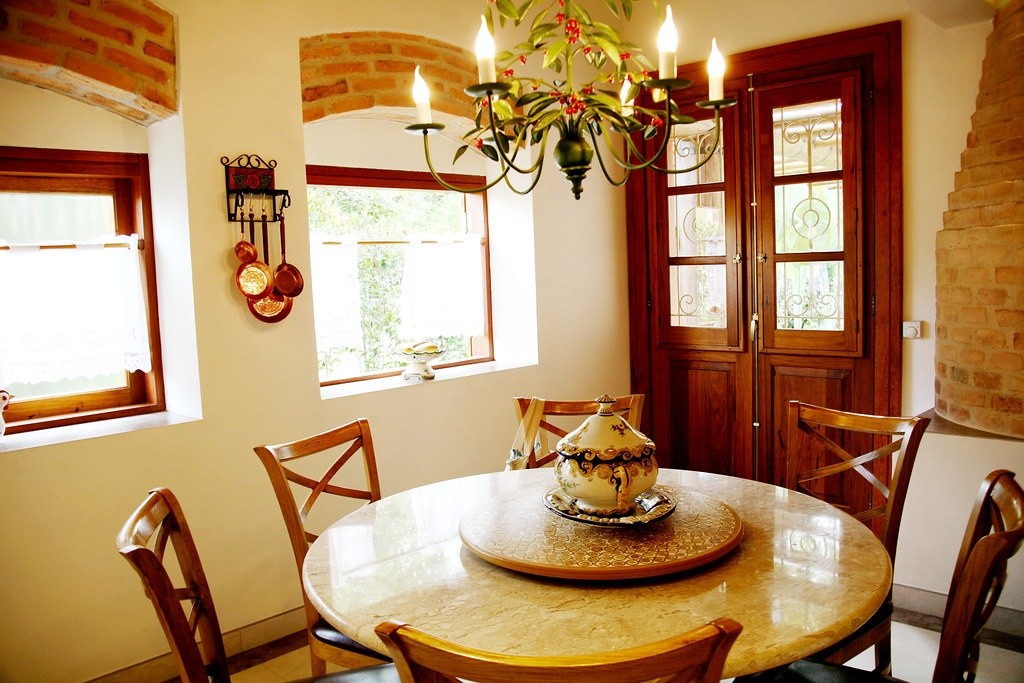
[{"x1": 555, "y1": 394, "x2": 658, "y2": 513}]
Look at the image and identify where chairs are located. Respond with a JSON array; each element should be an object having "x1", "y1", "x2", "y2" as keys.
[
  {"x1": 374, "y1": 615, "x2": 744, "y2": 683},
  {"x1": 732, "y1": 468, "x2": 1024, "y2": 683},
  {"x1": 115, "y1": 487, "x2": 401, "y2": 683},
  {"x1": 785, "y1": 399, "x2": 932, "y2": 678},
  {"x1": 252, "y1": 418, "x2": 394, "y2": 677},
  {"x1": 512, "y1": 393, "x2": 645, "y2": 469}
]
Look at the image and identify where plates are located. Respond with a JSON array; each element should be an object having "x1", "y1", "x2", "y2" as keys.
[{"x1": 542, "y1": 484, "x2": 676, "y2": 531}]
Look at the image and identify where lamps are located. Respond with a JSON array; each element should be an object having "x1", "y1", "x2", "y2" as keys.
[{"x1": 404, "y1": 0, "x2": 738, "y2": 201}]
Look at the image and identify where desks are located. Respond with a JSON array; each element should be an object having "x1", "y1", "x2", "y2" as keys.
[{"x1": 301, "y1": 467, "x2": 893, "y2": 683}]
